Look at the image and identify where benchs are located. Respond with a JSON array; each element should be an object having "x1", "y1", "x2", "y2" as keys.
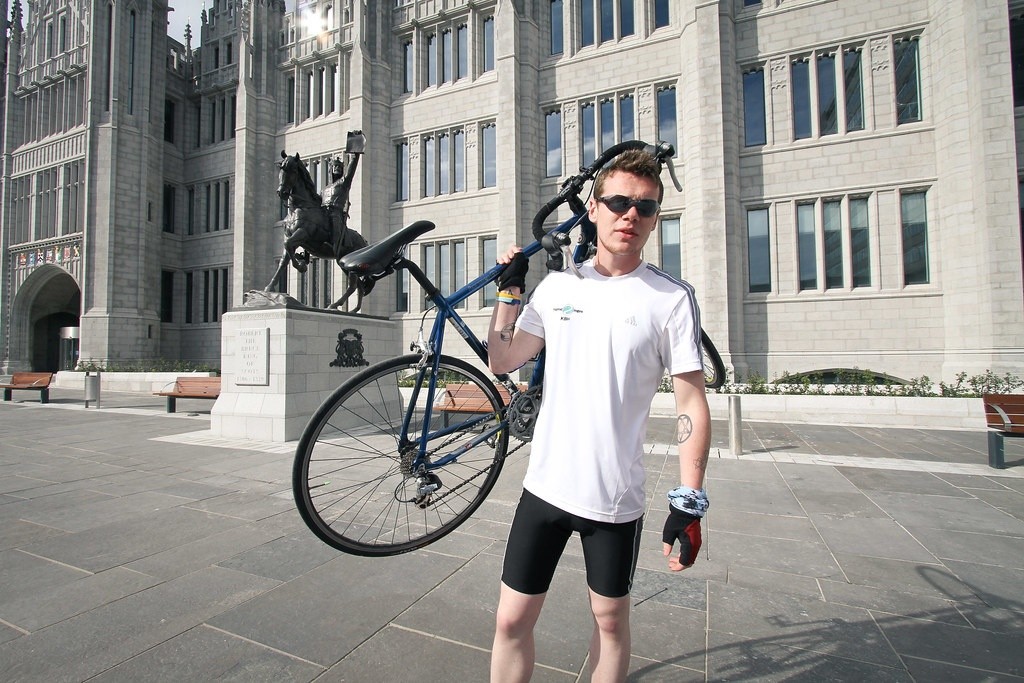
[
  {"x1": 432, "y1": 384, "x2": 528, "y2": 430},
  {"x1": 0, "y1": 372, "x2": 56, "y2": 403},
  {"x1": 153, "y1": 377, "x2": 221, "y2": 413},
  {"x1": 983, "y1": 394, "x2": 1024, "y2": 469}
]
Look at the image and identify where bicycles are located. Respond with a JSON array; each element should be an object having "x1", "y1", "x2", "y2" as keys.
[{"x1": 290, "y1": 140, "x2": 726, "y2": 557}]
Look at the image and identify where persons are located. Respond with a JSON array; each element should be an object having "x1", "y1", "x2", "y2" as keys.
[
  {"x1": 488, "y1": 151, "x2": 712, "y2": 683},
  {"x1": 295, "y1": 130, "x2": 363, "y2": 265}
]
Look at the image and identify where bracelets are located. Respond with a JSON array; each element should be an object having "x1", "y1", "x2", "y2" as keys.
[{"x1": 496, "y1": 292, "x2": 522, "y2": 304}]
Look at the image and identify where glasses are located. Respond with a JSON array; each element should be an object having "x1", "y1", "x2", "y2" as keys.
[{"x1": 596, "y1": 195, "x2": 661, "y2": 218}]
